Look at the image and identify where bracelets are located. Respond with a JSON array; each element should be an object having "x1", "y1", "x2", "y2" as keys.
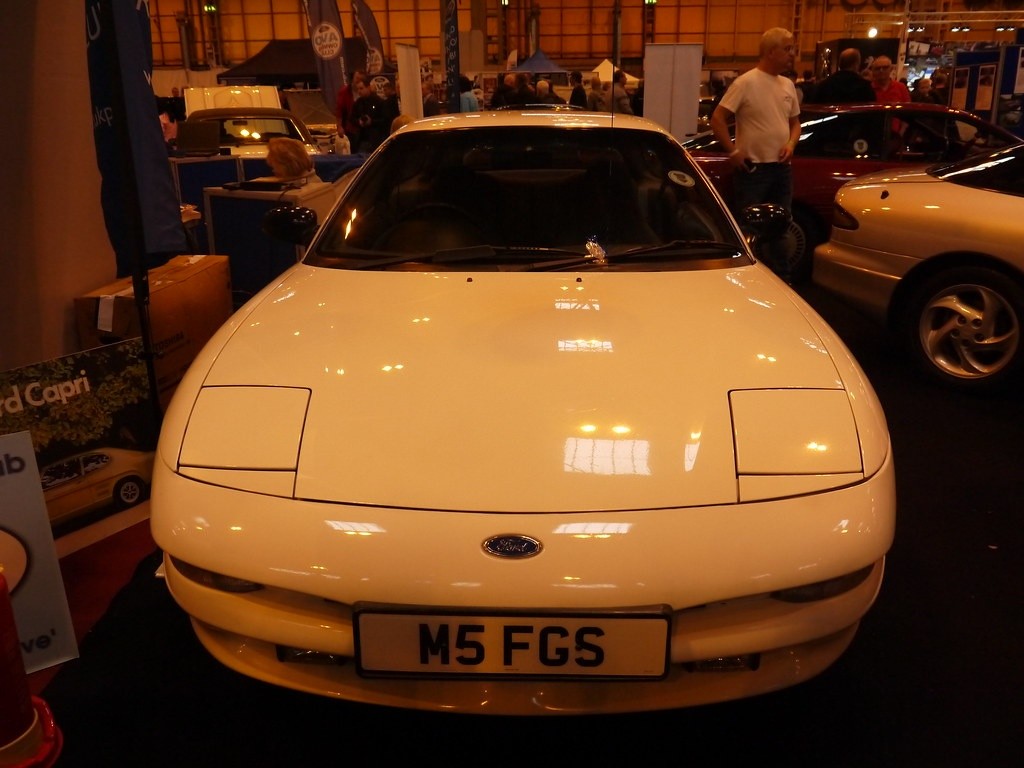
[{"x1": 729, "y1": 149, "x2": 739, "y2": 157}]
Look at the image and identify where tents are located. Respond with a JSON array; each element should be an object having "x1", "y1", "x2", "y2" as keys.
[
  {"x1": 216, "y1": 38, "x2": 367, "y2": 87},
  {"x1": 510, "y1": 51, "x2": 568, "y2": 85},
  {"x1": 582, "y1": 59, "x2": 639, "y2": 89}
]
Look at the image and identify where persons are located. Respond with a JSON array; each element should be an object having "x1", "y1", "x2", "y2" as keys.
[
  {"x1": 711, "y1": 27, "x2": 801, "y2": 281},
  {"x1": 873, "y1": 55, "x2": 948, "y2": 135},
  {"x1": 813, "y1": 48, "x2": 875, "y2": 157},
  {"x1": 422, "y1": 83, "x2": 441, "y2": 117},
  {"x1": 267, "y1": 138, "x2": 323, "y2": 182},
  {"x1": 798, "y1": 70, "x2": 813, "y2": 103},
  {"x1": 172, "y1": 88, "x2": 179, "y2": 97},
  {"x1": 787, "y1": 71, "x2": 798, "y2": 82},
  {"x1": 342, "y1": 69, "x2": 401, "y2": 153},
  {"x1": 455, "y1": 70, "x2": 634, "y2": 117},
  {"x1": 859, "y1": 64, "x2": 873, "y2": 81}
]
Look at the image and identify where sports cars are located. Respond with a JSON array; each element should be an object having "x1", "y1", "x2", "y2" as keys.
[
  {"x1": 684, "y1": 103, "x2": 1023, "y2": 277},
  {"x1": 168, "y1": 110, "x2": 324, "y2": 157},
  {"x1": 40, "y1": 447, "x2": 156, "y2": 530},
  {"x1": 813, "y1": 142, "x2": 1023, "y2": 394},
  {"x1": 150, "y1": 108, "x2": 898, "y2": 709}
]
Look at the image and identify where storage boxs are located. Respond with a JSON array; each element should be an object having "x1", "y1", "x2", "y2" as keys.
[{"x1": 74, "y1": 252, "x2": 235, "y2": 410}]
